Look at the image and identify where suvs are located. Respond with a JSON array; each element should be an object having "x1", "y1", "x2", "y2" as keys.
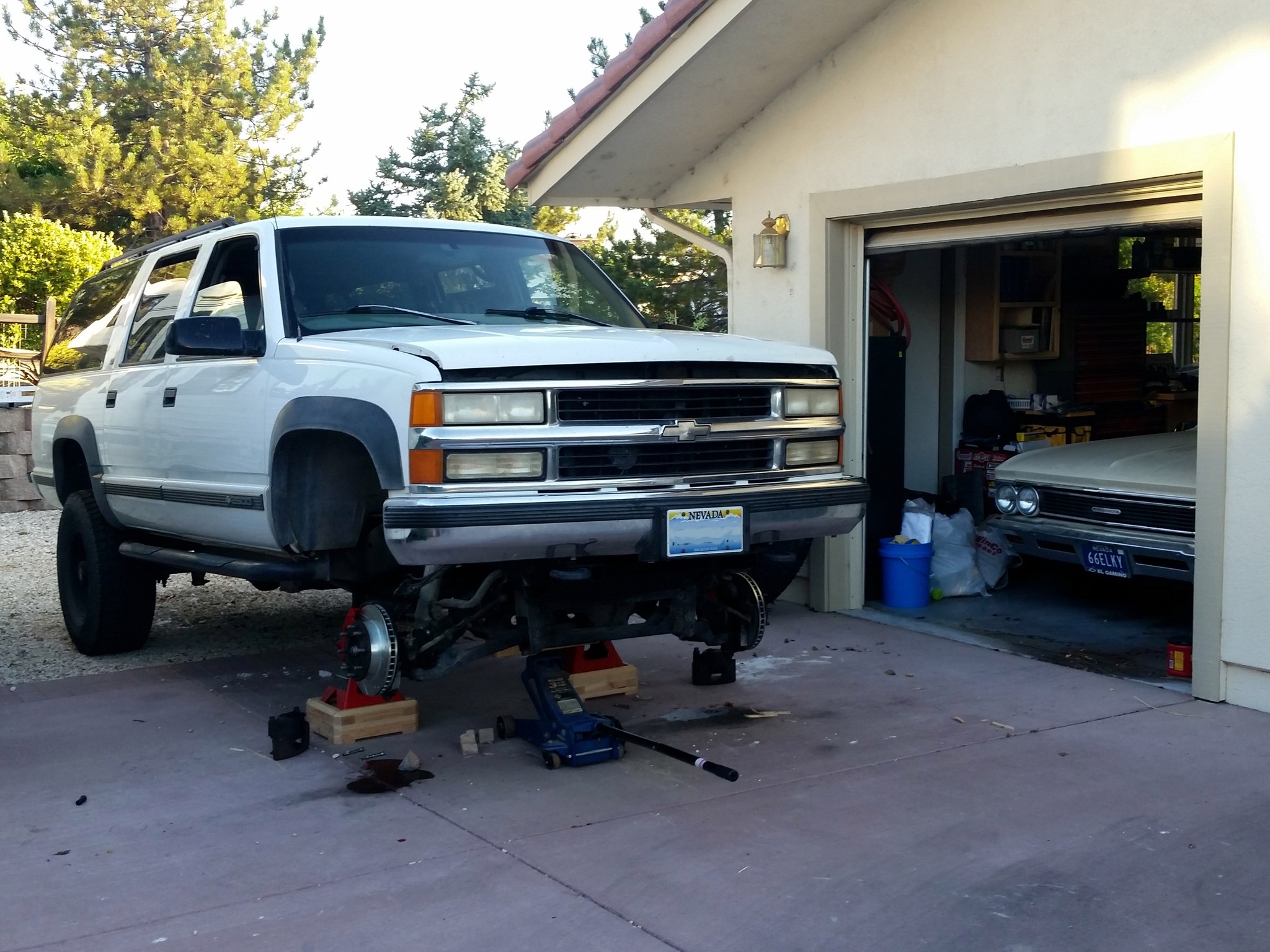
[{"x1": 26, "y1": 214, "x2": 870, "y2": 701}]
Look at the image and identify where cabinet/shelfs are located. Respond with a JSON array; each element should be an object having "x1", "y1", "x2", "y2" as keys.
[{"x1": 965, "y1": 250, "x2": 1061, "y2": 361}]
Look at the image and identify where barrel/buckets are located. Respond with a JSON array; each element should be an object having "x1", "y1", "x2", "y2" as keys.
[{"x1": 879, "y1": 537, "x2": 935, "y2": 607}]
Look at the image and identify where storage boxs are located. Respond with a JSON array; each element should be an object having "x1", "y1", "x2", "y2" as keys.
[{"x1": 1000, "y1": 326, "x2": 1040, "y2": 353}]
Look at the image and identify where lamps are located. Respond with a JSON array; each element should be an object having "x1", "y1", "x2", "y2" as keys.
[{"x1": 753, "y1": 211, "x2": 790, "y2": 269}]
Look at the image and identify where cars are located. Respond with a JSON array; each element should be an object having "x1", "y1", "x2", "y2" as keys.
[{"x1": 985, "y1": 423, "x2": 1199, "y2": 590}]
